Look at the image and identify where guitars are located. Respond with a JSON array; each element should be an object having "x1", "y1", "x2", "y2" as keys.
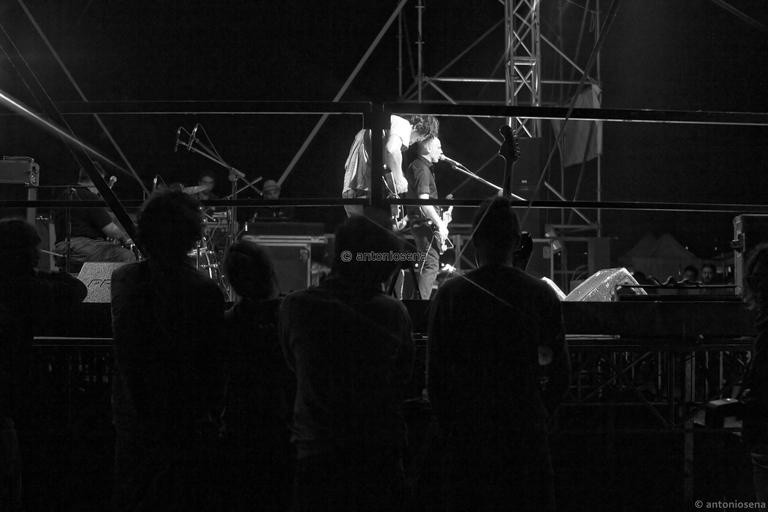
[
  {"x1": 475, "y1": 126, "x2": 534, "y2": 272},
  {"x1": 433, "y1": 194, "x2": 453, "y2": 252}
]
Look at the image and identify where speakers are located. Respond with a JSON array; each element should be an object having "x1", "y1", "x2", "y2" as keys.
[
  {"x1": 74, "y1": 261, "x2": 129, "y2": 337},
  {"x1": 563, "y1": 266, "x2": 647, "y2": 302},
  {"x1": 0, "y1": 155, "x2": 40, "y2": 223},
  {"x1": 731, "y1": 213, "x2": 768, "y2": 298}
]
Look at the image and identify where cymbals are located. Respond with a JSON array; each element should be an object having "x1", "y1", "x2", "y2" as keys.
[{"x1": 180, "y1": 185, "x2": 209, "y2": 194}]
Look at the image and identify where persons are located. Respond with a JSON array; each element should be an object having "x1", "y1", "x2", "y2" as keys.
[
  {"x1": 109, "y1": 189, "x2": 224, "y2": 511},
  {"x1": 701, "y1": 263, "x2": 719, "y2": 284},
  {"x1": 681, "y1": 266, "x2": 698, "y2": 283},
  {"x1": 0, "y1": 218, "x2": 89, "y2": 510},
  {"x1": 51, "y1": 161, "x2": 136, "y2": 262},
  {"x1": 276, "y1": 215, "x2": 415, "y2": 510},
  {"x1": 341, "y1": 114, "x2": 440, "y2": 298},
  {"x1": 193, "y1": 175, "x2": 222, "y2": 202},
  {"x1": 260, "y1": 178, "x2": 282, "y2": 199},
  {"x1": 425, "y1": 197, "x2": 566, "y2": 511},
  {"x1": 401, "y1": 135, "x2": 452, "y2": 297},
  {"x1": 218, "y1": 239, "x2": 298, "y2": 511}
]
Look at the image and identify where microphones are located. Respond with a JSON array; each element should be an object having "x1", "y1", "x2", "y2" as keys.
[
  {"x1": 186, "y1": 124, "x2": 199, "y2": 150},
  {"x1": 108, "y1": 175, "x2": 117, "y2": 189},
  {"x1": 174, "y1": 127, "x2": 182, "y2": 152},
  {"x1": 152, "y1": 177, "x2": 157, "y2": 190},
  {"x1": 440, "y1": 154, "x2": 464, "y2": 169}
]
